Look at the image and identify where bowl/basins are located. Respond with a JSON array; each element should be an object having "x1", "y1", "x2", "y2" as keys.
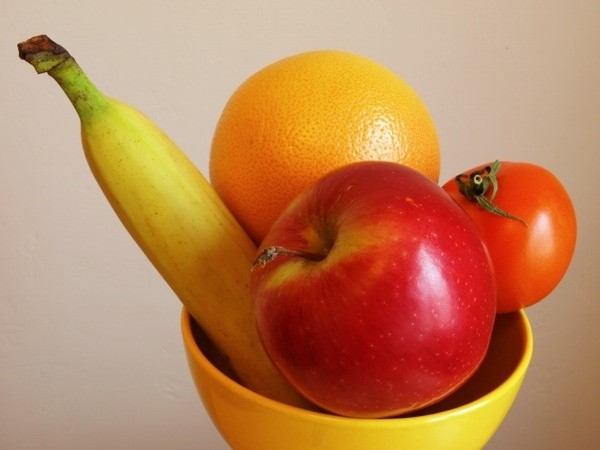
[{"x1": 181, "y1": 302, "x2": 536, "y2": 450}]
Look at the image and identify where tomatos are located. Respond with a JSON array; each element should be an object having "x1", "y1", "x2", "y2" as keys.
[{"x1": 441, "y1": 163, "x2": 576, "y2": 314}]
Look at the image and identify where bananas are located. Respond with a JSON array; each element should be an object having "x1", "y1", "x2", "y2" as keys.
[{"x1": 16, "y1": 35, "x2": 312, "y2": 409}]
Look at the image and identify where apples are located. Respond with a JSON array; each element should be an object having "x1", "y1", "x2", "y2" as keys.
[{"x1": 251, "y1": 162, "x2": 496, "y2": 419}]
